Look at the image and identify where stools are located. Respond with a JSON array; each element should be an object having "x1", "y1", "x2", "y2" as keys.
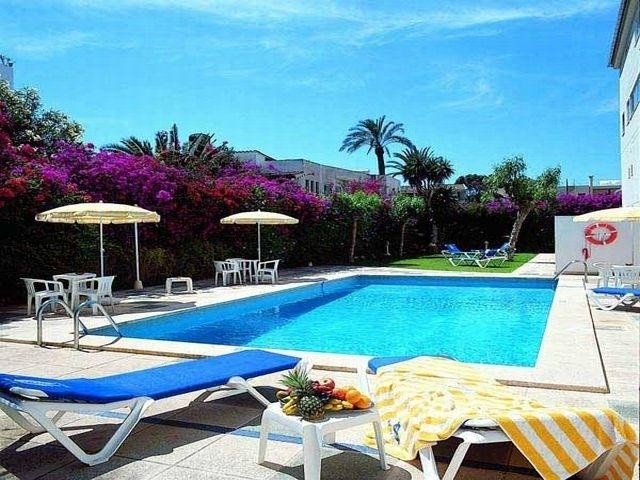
[{"x1": 166, "y1": 277, "x2": 193, "y2": 293}]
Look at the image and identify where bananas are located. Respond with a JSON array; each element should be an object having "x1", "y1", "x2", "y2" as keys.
[{"x1": 283, "y1": 398, "x2": 301, "y2": 416}]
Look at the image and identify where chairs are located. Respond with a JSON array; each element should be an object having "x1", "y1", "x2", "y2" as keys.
[
  {"x1": 70, "y1": 276, "x2": 116, "y2": 315},
  {"x1": 64, "y1": 273, "x2": 96, "y2": 309},
  {"x1": 441, "y1": 249, "x2": 490, "y2": 269},
  {"x1": 593, "y1": 261, "x2": 615, "y2": 287},
  {"x1": 357, "y1": 356, "x2": 624, "y2": 480},
  {"x1": 443, "y1": 244, "x2": 485, "y2": 258},
  {"x1": 20, "y1": 278, "x2": 68, "y2": 317},
  {"x1": 587, "y1": 287, "x2": 640, "y2": 311},
  {"x1": 255, "y1": 259, "x2": 280, "y2": 285},
  {"x1": 213, "y1": 261, "x2": 242, "y2": 287},
  {"x1": 610, "y1": 264, "x2": 639, "y2": 287},
  {"x1": 4, "y1": 350, "x2": 302, "y2": 465}
]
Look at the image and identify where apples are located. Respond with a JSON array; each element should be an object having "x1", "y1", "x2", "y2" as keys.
[
  {"x1": 332, "y1": 389, "x2": 344, "y2": 399},
  {"x1": 320, "y1": 378, "x2": 334, "y2": 390}
]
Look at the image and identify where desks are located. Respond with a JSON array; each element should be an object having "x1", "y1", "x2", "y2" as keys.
[
  {"x1": 258, "y1": 403, "x2": 392, "y2": 480},
  {"x1": 225, "y1": 257, "x2": 252, "y2": 285},
  {"x1": 51, "y1": 273, "x2": 97, "y2": 313}
]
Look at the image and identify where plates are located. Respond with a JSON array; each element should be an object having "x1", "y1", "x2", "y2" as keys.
[{"x1": 326, "y1": 400, "x2": 375, "y2": 414}]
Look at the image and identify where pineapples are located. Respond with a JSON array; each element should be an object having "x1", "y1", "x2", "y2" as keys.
[{"x1": 276, "y1": 364, "x2": 324, "y2": 419}]
